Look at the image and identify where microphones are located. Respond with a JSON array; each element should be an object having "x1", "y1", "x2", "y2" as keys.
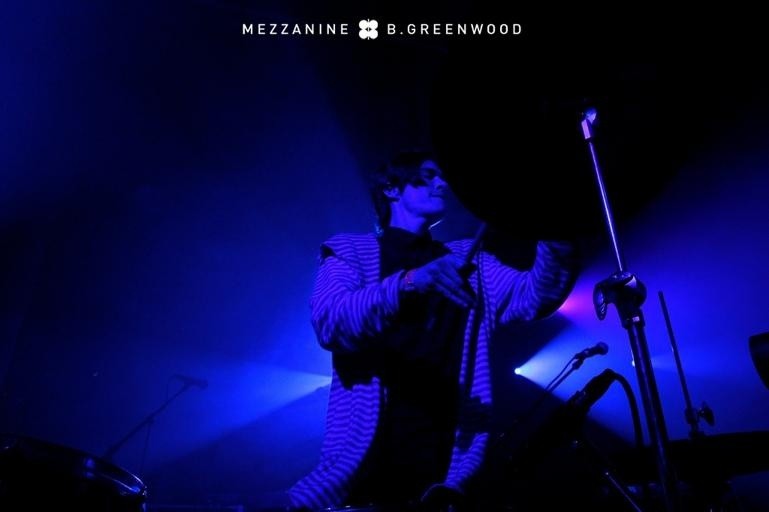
[
  {"x1": 571, "y1": 341, "x2": 609, "y2": 359},
  {"x1": 176, "y1": 372, "x2": 211, "y2": 390}
]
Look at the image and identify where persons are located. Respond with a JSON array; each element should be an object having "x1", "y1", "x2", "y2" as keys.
[{"x1": 288, "y1": 151, "x2": 585, "y2": 510}]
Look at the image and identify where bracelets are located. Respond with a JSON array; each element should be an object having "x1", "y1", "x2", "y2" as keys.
[{"x1": 405, "y1": 268, "x2": 414, "y2": 291}]
